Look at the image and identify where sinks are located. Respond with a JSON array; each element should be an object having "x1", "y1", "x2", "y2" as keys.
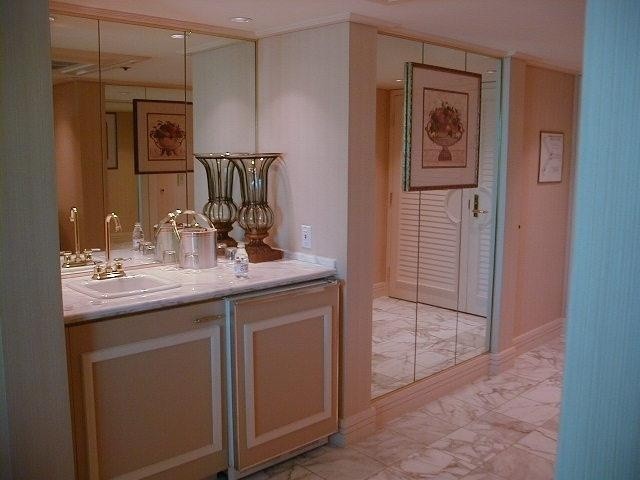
[
  {"x1": 65, "y1": 272, "x2": 183, "y2": 301},
  {"x1": 59, "y1": 254, "x2": 116, "y2": 276}
]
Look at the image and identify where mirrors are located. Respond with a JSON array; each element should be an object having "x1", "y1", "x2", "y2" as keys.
[
  {"x1": 370, "y1": 33, "x2": 503, "y2": 401},
  {"x1": 48, "y1": 11, "x2": 258, "y2": 275}
]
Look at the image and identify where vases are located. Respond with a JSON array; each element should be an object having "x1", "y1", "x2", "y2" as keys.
[
  {"x1": 193, "y1": 150, "x2": 240, "y2": 254},
  {"x1": 225, "y1": 150, "x2": 287, "y2": 262}
]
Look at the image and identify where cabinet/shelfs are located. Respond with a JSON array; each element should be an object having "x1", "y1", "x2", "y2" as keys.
[
  {"x1": 65, "y1": 284, "x2": 340, "y2": 480},
  {"x1": 387, "y1": 80, "x2": 497, "y2": 318}
]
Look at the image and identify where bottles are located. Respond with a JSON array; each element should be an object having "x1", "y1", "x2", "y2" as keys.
[
  {"x1": 233, "y1": 243, "x2": 249, "y2": 280},
  {"x1": 132, "y1": 223, "x2": 145, "y2": 250}
]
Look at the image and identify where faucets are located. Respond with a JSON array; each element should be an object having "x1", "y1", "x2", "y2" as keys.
[
  {"x1": 68, "y1": 206, "x2": 81, "y2": 256},
  {"x1": 104, "y1": 211, "x2": 121, "y2": 264}
]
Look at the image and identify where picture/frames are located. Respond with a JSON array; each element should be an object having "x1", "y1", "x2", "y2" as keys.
[
  {"x1": 537, "y1": 129, "x2": 566, "y2": 184},
  {"x1": 405, "y1": 57, "x2": 481, "y2": 191},
  {"x1": 103, "y1": 108, "x2": 121, "y2": 173},
  {"x1": 129, "y1": 95, "x2": 193, "y2": 176}
]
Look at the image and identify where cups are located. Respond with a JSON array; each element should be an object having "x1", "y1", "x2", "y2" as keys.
[
  {"x1": 162, "y1": 251, "x2": 180, "y2": 271},
  {"x1": 139, "y1": 241, "x2": 160, "y2": 264},
  {"x1": 184, "y1": 250, "x2": 199, "y2": 273}
]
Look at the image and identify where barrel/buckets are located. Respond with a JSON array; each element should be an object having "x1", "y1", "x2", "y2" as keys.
[
  {"x1": 152, "y1": 213, "x2": 186, "y2": 263},
  {"x1": 178, "y1": 210, "x2": 218, "y2": 270}
]
